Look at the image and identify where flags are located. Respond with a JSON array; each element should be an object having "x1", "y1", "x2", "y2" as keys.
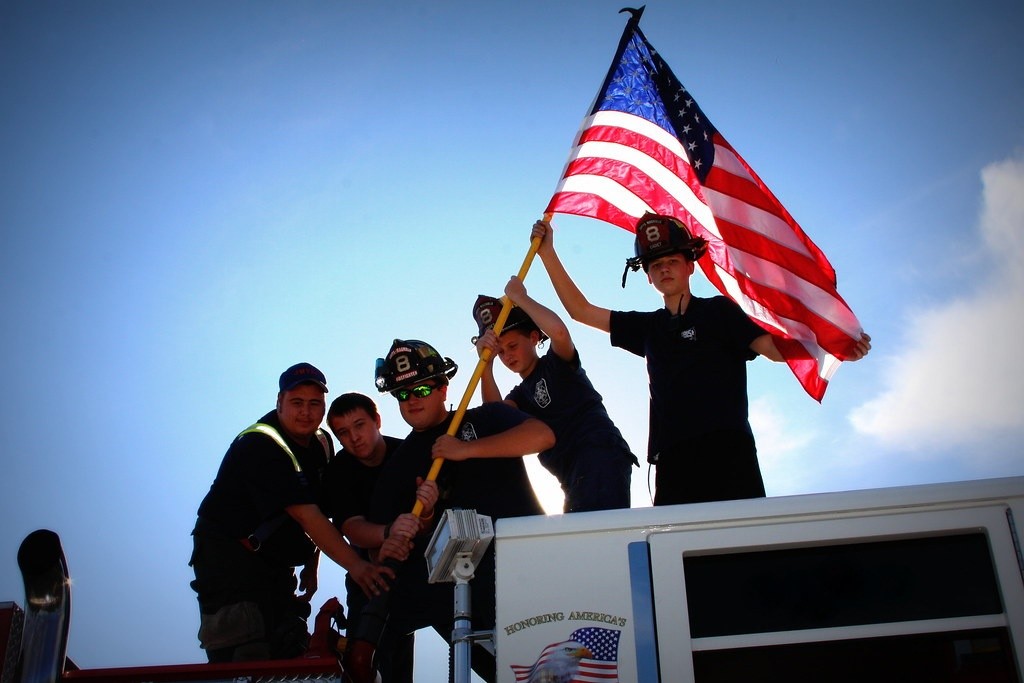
[{"x1": 548, "y1": 4, "x2": 862, "y2": 405}]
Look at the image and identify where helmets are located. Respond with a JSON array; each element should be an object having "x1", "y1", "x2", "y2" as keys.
[
  {"x1": 472, "y1": 293, "x2": 549, "y2": 343},
  {"x1": 621, "y1": 209, "x2": 709, "y2": 270},
  {"x1": 373, "y1": 339, "x2": 458, "y2": 392}
]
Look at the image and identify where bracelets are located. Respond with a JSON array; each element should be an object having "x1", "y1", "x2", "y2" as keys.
[{"x1": 384, "y1": 524, "x2": 391, "y2": 539}]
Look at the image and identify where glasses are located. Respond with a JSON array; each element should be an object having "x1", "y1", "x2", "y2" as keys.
[{"x1": 394, "y1": 380, "x2": 445, "y2": 402}]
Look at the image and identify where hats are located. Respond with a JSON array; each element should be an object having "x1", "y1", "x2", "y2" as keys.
[{"x1": 280, "y1": 362, "x2": 328, "y2": 394}]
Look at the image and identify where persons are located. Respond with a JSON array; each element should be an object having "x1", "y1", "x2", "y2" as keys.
[
  {"x1": 529, "y1": 209, "x2": 871, "y2": 506},
  {"x1": 473, "y1": 274, "x2": 642, "y2": 514},
  {"x1": 188, "y1": 363, "x2": 394, "y2": 666},
  {"x1": 376, "y1": 336, "x2": 557, "y2": 522},
  {"x1": 322, "y1": 394, "x2": 496, "y2": 683}
]
